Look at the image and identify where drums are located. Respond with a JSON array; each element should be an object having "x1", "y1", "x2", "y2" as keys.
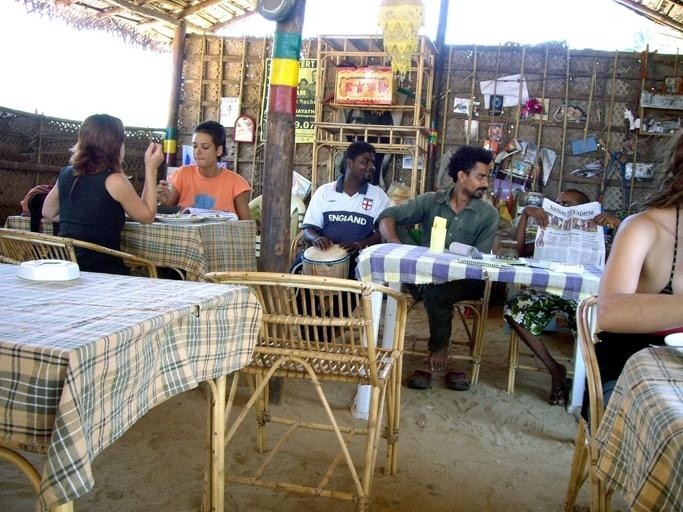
[{"x1": 302, "y1": 244, "x2": 350, "y2": 338}]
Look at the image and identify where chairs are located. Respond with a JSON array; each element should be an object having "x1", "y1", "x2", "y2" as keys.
[
  {"x1": 0, "y1": 228, "x2": 156, "y2": 279},
  {"x1": 556, "y1": 294, "x2": 619, "y2": 510},
  {"x1": 193, "y1": 267, "x2": 407, "y2": 512}
]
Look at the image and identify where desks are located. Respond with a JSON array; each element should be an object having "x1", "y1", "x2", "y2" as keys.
[
  {"x1": 0, "y1": 263, "x2": 262, "y2": 512},
  {"x1": 350, "y1": 240, "x2": 607, "y2": 428},
  {"x1": 0, "y1": 213, "x2": 256, "y2": 285}
]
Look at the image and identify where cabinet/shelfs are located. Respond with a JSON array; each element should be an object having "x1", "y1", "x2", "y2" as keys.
[{"x1": 308, "y1": 35, "x2": 441, "y2": 231}]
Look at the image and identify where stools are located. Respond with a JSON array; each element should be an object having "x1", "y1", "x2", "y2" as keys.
[{"x1": 392, "y1": 278, "x2": 576, "y2": 399}]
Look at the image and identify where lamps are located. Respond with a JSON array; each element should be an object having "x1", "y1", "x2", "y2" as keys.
[{"x1": 378, "y1": 0, "x2": 423, "y2": 86}]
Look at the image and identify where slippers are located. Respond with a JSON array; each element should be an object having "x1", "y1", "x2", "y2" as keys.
[
  {"x1": 406, "y1": 370, "x2": 432, "y2": 390},
  {"x1": 446, "y1": 371, "x2": 470, "y2": 391}
]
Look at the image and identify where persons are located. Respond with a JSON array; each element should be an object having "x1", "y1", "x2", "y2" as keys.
[
  {"x1": 503, "y1": 189, "x2": 622, "y2": 407},
  {"x1": 586, "y1": 126, "x2": 683, "y2": 435},
  {"x1": 157, "y1": 120, "x2": 252, "y2": 220},
  {"x1": 290, "y1": 141, "x2": 391, "y2": 341},
  {"x1": 378, "y1": 147, "x2": 499, "y2": 372},
  {"x1": 41, "y1": 114, "x2": 163, "y2": 274}
]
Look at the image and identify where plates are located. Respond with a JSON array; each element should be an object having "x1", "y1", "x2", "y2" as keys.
[
  {"x1": 663, "y1": 332, "x2": 683, "y2": 356},
  {"x1": 154, "y1": 212, "x2": 234, "y2": 223}
]
